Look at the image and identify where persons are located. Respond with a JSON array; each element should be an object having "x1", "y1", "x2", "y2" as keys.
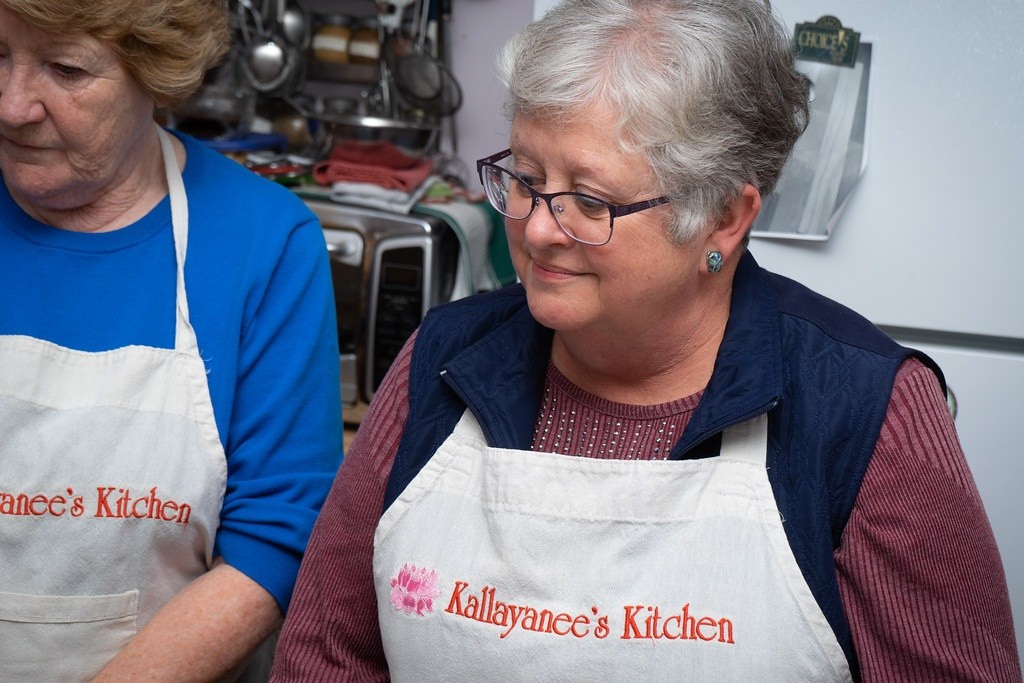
[
  {"x1": 0, "y1": 0, "x2": 345, "y2": 683},
  {"x1": 263, "y1": 2, "x2": 1024, "y2": 683}
]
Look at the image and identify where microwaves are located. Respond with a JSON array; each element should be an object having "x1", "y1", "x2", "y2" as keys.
[{"x1": 285, "y1": 185, "x2": 463, "y2": 429}]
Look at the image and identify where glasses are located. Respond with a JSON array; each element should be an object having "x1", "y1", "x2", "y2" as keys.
[{"x1": 476, "y1": 151, "x2": 719, "y2": 245}]
